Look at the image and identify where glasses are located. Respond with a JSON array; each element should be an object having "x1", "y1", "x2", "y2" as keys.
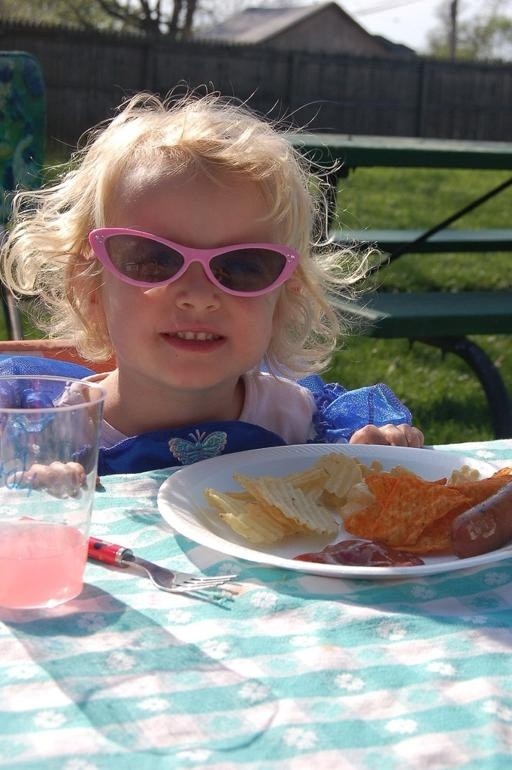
[{"x1": 89, "y1": 227, "x2": 302, "y2": 297}]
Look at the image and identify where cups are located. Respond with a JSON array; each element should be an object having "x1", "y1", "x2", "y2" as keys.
[{"x1": 0, "y1": 374, "x2": 108, "y2": 612}]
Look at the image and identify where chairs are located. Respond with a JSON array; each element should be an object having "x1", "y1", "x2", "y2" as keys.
[{"x1": 1, "y1": 338, "x2": 118, "y2": 373}]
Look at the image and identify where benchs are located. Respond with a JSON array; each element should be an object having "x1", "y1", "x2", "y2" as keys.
[{"x1": 278, "y1": 224, "x2": 512, "y2": 433}]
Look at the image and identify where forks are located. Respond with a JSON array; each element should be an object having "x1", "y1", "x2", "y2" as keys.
[{"x1": 87, "y1": 537, "x2": 241, "y2": 594}]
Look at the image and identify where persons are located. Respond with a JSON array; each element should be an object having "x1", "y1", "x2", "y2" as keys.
[{"x1": 0, "y1": 80, "x2": 425, "y2": 488}]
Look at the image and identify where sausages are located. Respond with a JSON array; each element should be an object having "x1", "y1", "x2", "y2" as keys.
[{"x1": 452, "y1": 482, "x2": 511, "y2": 560}]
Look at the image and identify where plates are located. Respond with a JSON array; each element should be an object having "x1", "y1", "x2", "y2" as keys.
[{"x1": 156, "y1": 442, "x2": 512, "y2": 579}]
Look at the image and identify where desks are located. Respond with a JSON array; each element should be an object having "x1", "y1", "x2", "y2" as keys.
[{"x1": 278, "y1": 129, "x2": 512, "y2": 194}]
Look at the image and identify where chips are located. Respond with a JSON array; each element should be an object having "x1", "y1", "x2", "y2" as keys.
[{"x1": 204, "y1": 451, "x2": 511, "y2": 554}]
